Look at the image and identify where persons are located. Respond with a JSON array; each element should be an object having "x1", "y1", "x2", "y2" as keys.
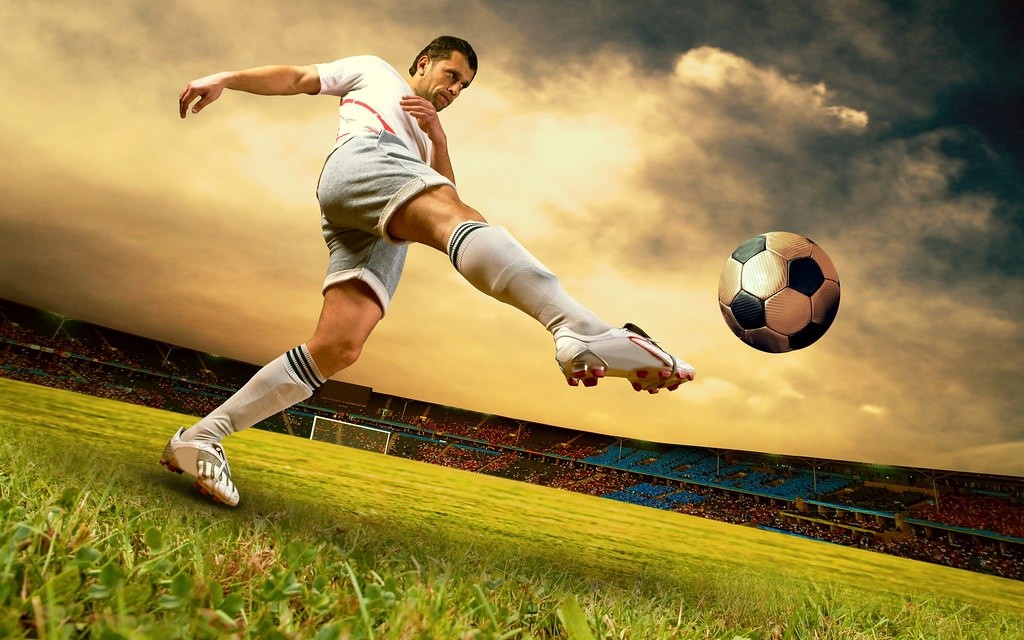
[{"x1": 158, "y1": 35, "x2": 695, "y2": 505}]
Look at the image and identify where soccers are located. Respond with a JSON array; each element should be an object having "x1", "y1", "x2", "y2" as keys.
[{"x1": 716, "y1": 230, "x2": 844, "y2": 353}]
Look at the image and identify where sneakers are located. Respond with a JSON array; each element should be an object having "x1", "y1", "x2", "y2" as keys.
[
  {"x1": 553, "y1": 321, "x2": 696, "y2": 394},
  {"x1": 159, "y1": 426, "x2": 240, "y2": 507}
]
